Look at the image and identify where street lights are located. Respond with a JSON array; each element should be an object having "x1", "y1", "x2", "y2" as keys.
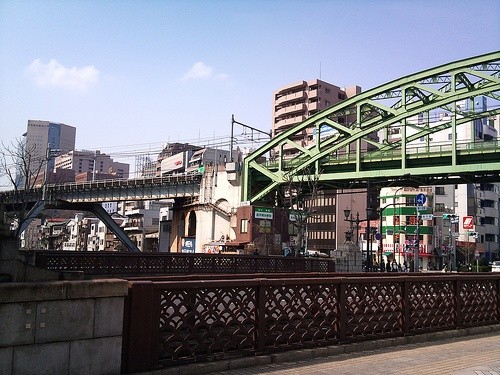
[{"x1": 343, "y1": 205, "x2": 381, "y2": 245}]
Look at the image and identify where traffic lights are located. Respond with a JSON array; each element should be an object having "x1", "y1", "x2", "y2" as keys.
[
  {"x1": 413, "y1": 240, "x2": 419, "y2": 249},
  {"x1": 443, "y1": 214, "x2": 455, "y2": 220},
  {"x1": 467, "y1": 232, "x2": 476, "y2": 236}
]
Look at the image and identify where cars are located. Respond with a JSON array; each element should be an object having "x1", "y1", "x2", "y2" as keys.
[{"x1": 492, "y1": 261, "x2": 500, "y2": 272}]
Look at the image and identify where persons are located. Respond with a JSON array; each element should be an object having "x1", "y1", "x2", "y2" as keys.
[
  {"x1": 363, "y1": 258, "x2": 414, "y2": 272},
  {"x1": 217, "y1": 235, "x2": 231, "y2": 252},
  {"x1": 456, "y1": 260, "x2": 462, "y2": 272},
  {"x1": 311, "y1": 252, "x2": 320, "y2": 257},
  {"x1": 251, "y1": 248, "x2": 260, "y2": 256},
  {"x1": 444, "y1": 265, "x2": 448, "y2": 272},
  {"x1": 285, "y1": 250, "x2": 292, "y2": 257},
  {"x1": 466, "y1": 263, "x2": 472, "y2": 272}
]
